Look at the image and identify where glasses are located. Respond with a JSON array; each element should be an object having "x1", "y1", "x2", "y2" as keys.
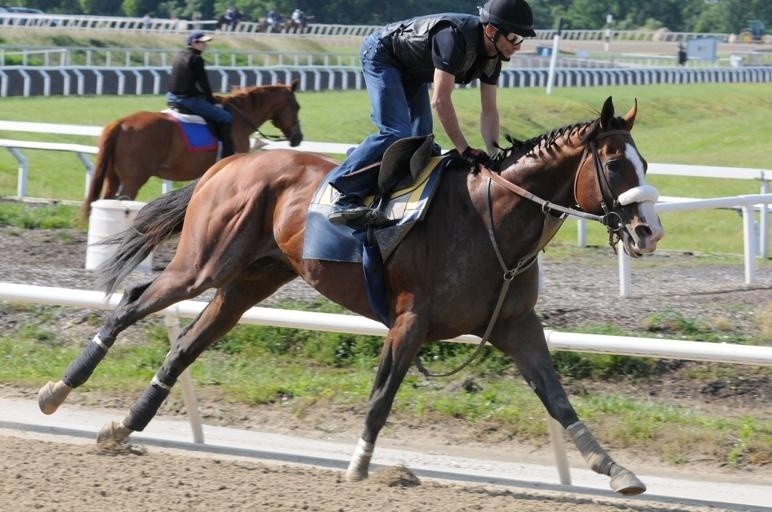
[{"x1": 489, "y1": 23, "x2": 524, "y2": 45}]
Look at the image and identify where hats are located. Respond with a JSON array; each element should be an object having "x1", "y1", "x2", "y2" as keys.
[
  {"x1": 478, "y1": 0, "x2": 537, "y2": 39},
  {"x1": 186, "y1": 31, "x2": 213, "y2": 46}
]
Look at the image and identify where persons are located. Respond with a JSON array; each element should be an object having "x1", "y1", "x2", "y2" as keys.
[
  {"x1": 167, "y1": 30, "x2": 235, "y2": 158},
  {"x1": 327, "y1": 0, "x2": 537, "y2": 226},
  {"x1": 140, "y1": 8, "x2": 304, "y2": 31},
  {"x1": 676, "y1": 42, "x2": 688, "y2": 68}
]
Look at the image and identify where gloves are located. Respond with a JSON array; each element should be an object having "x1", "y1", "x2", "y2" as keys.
[{"x1": 460, "y1": 145, "x2": 490, "y2": 165}]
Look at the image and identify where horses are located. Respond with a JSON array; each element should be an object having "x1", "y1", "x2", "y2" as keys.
[
  {"x1": 80, "y1": 77, "x2": 304, "y2": 231},
  {"x1": 35, "y1": 95, "x2": 666, "y2": 497},
  {"x1": 214, "y1": 11, "x2": 316, "y2": 33}
]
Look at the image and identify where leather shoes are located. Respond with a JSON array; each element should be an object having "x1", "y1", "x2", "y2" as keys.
[{"x1": 327, "y1": 196, "x2": 372, "y2": 225}]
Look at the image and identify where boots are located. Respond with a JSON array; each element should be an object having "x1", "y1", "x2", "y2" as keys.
[{"x1": 220, "y1": 121, "x2": 236, "y2": 158}]
